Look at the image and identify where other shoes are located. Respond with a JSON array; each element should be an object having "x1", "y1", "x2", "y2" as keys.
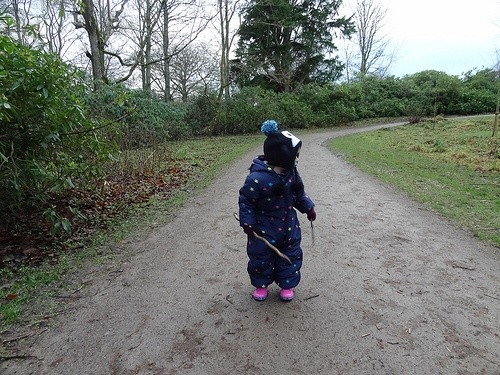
[
  {"x1": 280, "y1": 289, "x2": 294, "y2": 300},
  {"x1": 254, "y1": 288, "x2": 268, "y2": 300}
]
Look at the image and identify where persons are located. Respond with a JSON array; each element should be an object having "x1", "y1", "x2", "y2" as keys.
[{"x1": 238, "y1": 120, "x2": 317, "y2": 301}]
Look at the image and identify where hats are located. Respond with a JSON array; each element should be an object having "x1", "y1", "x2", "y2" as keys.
[{"x1": 261, "y1": 120, "x2": 302, "y2": 196}]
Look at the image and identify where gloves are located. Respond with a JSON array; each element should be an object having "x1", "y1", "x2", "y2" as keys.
[
  {"x1": 243, "y1": 225, "x2": 257, "y2": 235},
  {"x1": 306, "y1": 207, "x2": 316, "y2": 221}
]
